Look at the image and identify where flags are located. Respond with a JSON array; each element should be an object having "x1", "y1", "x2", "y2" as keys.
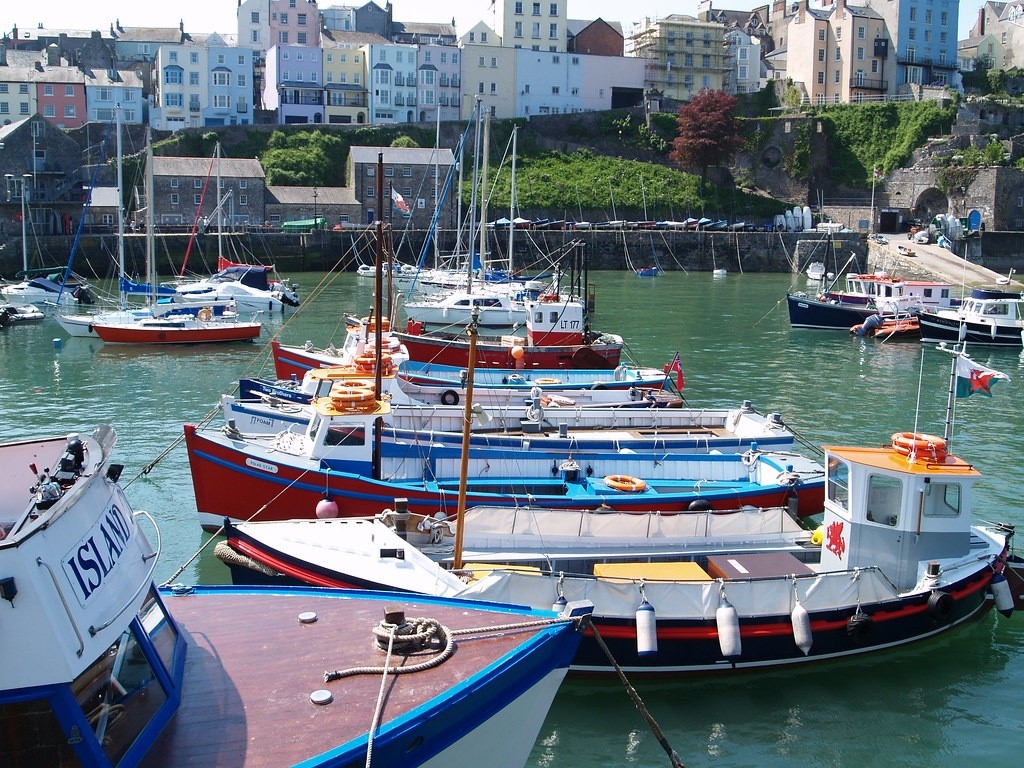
[
  {"x1": 956, "y1": 354, "x2": 1011, "y2": 399},
  {"x1": 671, "y1": 352, "x2": 684, "y2": 392},
  {"x1": 391, "y1": 186, "x2": 409, "y2": 213}
]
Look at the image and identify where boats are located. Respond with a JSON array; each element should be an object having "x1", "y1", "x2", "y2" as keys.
[
  {"x1": 875, "y1": 235, "x2": 889, "y2": 245},
  {"x1": 897, "y1": 237, "x2": 929, "y2": 256},
  {"x1": 786, "y1": 273, "x2": 998, "y2": 339},
  {"x1": 214, "y1": 432, "x2": 1016, "y2": 682},
  {"x1": 1, "y1": 278, "x2": 96, "y2": 305},
  {"x1": 713, "y1": 269, "x2": 727, "y2": 276},
  {"x1": 0, "y1": 423, "x2": 595, "y2": 768},
  {"x1": 636, "y1": 267, "x2": 658, "y2": 275},
  {"x1": 485, "y1": 217, "x2": 765, "y2": 231},
  {"x1": 160, "y1": 267, "x2": 301, "y2": 313},
  {"x1": 773, "y1": 206, "x2": 812, "y2": 232},
  {"x1": 183, "y1": 239, "x2": 826, "y2": 538},
  {"x1": 907, "y1": 287, "x2": 1024, "y2": 346},
  {"x1": 0, "y1": 293, "x2": 44, "y2": 324}
]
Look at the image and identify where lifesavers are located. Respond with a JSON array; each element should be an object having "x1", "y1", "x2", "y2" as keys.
[
  {"x1": 534, "y1": 378, "x2": 562, "y2": 384},
  {"x1": 892, "y1": 443, "x2": 946, "y2": 459},
  {"x1": 603, "y1": 475, "x2": 646, "y2": 491},
  {"x1": 368, "y1": 337, "x2": 400, "y2": 353},
  {"x1": 891, "y1": 432, "x2": 946, "y2": 450},
  {"x1": 537, "y1": 297, "x2": 560, "y2": 302},
  {"x1": 353, "y1": 353, "x2": 393, "y2": 375},
  {"x1": 847, "y1": 614, "x2": 873, "y2": 644},
  {"x1": 540, "y1": 294, "x2": 559, "y2": 298},
  {"x1": 198, "y1": 309, "x2": 211, "y2": 321},
  {"x1": 329, "y1": 379, "x2": 377, "y2": 412},
  {"x1": 369, "y1": 316, "x2": 389, "y2": 330},
  {"x1": 441, "y1": 390, "x2": 459, "y2": 405},
  {"x1": 928, "y1": 592, "x2": 955, "y2": 619},
  {"x1": 688, "y1": 500, "x2": 712, "y2": 511}
]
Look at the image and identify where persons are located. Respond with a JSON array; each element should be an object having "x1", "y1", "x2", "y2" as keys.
[
  {"x1": 139, "y1": 220, "x2": 145, "y2": 234},
  {"x1": 130, "y1": 220, "x2": 137, "y2": 233},
  {"x1": 204, "y1": 216, "x2": 210, "y2": 235},
  {"x1": 197, "y1": 216, "x2": 204, "y2": 233}
]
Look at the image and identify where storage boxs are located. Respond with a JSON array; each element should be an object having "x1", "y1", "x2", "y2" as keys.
[{"x1": 501, "y1": 335, "x2": 525, "y2": 347}]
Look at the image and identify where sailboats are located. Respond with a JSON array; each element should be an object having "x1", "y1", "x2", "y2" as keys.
[
  {"x1": 173, "y1": 140, "x2": 289, "y2": 284},
  {"x1": 85, "y1": 125, "x2": 264, "y2": 344},
  {"x1": 2, "y1": 179, "x2": 87, "y2": 288},
  {"x1": 356, "y1": 99, "x2": 587, "y2": 325},
  {"x1": 44, "y1": 102, "x2": 240, "y2": 338}
]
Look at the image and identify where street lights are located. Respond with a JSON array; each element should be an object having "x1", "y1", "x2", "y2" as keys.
[{"x1": 312, "y1": 186, "x2": 318, "y2": 229}]
